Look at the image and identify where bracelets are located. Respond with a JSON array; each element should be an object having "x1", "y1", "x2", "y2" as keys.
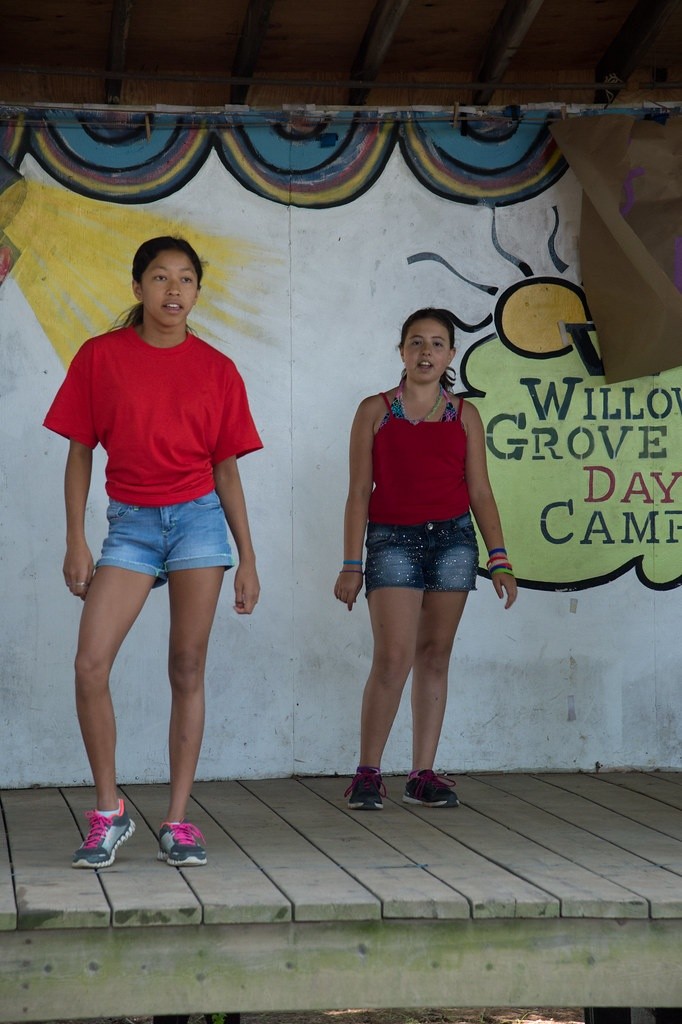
[
  {"x1": 343, "y1": 560, "x2": 363, "y2": 565},
  {"x1": 338, "y1": 570, "x2": 365, "y2": 576},
  {"x1": 486, "y1": 548, "x2": 515, "y2": 577}
]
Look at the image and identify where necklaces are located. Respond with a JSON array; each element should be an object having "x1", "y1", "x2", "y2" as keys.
[{"x1": 399, "y1": 379, "x2": 443, "y2": 426}]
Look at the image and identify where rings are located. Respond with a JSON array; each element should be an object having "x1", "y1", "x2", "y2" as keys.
[{"x1": 75, "y1": 582, "x2": 87, "y2": 586}]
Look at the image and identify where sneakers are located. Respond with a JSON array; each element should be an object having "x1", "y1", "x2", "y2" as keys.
[
  {"x1": 71, "y1": 799, "x2": 136, "y2": 868},
  {"x1": 157, "y1": 819, "x2": 208, "y2": 866},
  {"x1": 402, "y1": 770, "x2": 460, "y2": 808},
  {"x1": 348, "y1": 768, "x2": 384, "y2": 810}
]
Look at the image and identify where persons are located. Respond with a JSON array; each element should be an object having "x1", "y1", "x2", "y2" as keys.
[
  {"x1": 334, "y1": 307, "x2": 519, "y2": 813},
  {"x1": 41, "y1": 234, "x2": 264, "y2": 867}
]
